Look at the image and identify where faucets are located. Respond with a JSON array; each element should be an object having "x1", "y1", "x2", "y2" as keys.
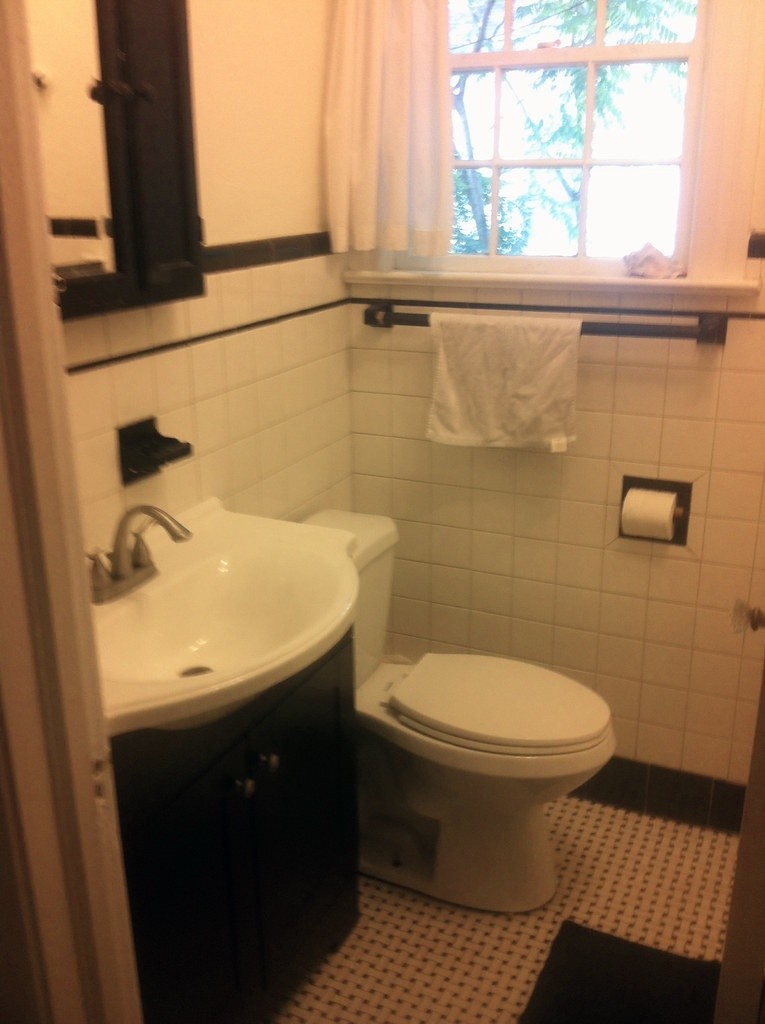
[{"x1": 84, "y1": 502, "x2": 193, "y2": 606}]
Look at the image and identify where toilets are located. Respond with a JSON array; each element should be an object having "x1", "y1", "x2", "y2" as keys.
[{"x1": 293, "y1": 505, "x2": 619, "y2": 918}]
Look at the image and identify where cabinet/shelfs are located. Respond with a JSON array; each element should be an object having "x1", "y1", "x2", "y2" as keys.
[{"x1": 109, "y1": 617, "x2": 361, "y2": 1024}]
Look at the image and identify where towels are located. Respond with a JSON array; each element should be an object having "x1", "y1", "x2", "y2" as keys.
[{"x1": 420, "y1": 310, "x2": 586, "y2": 454}]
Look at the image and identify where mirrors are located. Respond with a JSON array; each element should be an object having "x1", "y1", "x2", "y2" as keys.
[{"x1": 25, "y1": 0, "x2": 119, "y2": 281}]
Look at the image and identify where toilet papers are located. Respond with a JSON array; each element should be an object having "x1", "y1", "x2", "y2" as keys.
[{"x1": 618, "y1": 486, "x2": 682, "y2": 541}]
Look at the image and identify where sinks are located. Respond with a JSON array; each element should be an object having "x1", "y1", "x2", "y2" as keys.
[{"x1": 83, "y1": 493, "x2": 364, "y2": 736}]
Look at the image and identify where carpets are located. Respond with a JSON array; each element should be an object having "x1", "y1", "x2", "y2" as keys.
[{"x1": 516, "y1": 919, "x2": 721, "y2": 1024}]
